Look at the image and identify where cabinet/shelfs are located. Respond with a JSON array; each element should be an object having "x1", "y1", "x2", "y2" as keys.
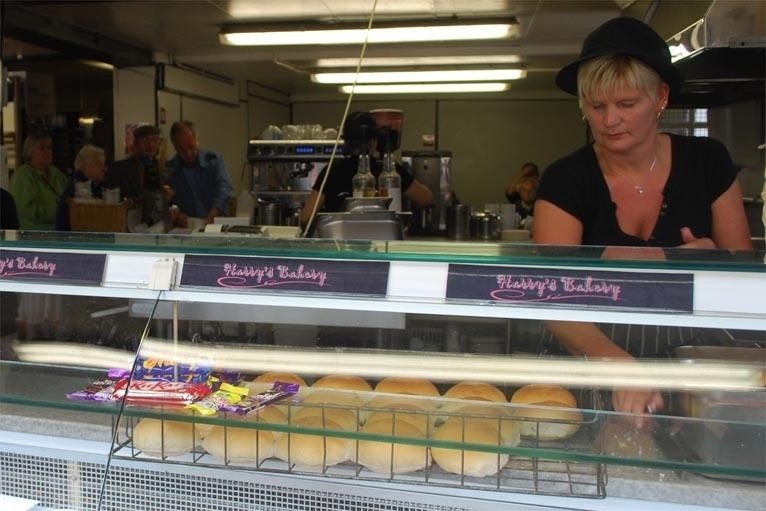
[
  {"x1": 246, "y1": 138, "x2": 452, "y2": 237},
  {"x1": 0, "y1": 229, "x2": 765, "y2": 511}
]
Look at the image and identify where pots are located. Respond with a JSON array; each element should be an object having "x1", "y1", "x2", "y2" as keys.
[{"x1": 469, "y1": 208, "x2": 502, "y2": 241}]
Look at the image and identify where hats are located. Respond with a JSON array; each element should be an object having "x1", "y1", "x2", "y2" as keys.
[
  {"x1": 133, "y1": 125, "x2": 157, "y2": 139},
  {"x1": 556, "y1": 16, "x2": 684, "y2": 100},
  {"x1": 342, "y1": 111, "x2": 387, "y2": 155}
]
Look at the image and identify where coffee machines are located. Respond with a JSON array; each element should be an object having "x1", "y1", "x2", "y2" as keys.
[
  {"x1": 402, "y1": 147, "x2": 454, "y2": 238},
  {"x1": 246, "y1": 138, "x2": 347, "y2": 226}
]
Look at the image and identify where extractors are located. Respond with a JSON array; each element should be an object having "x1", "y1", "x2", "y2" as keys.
[{"x1": 615, "y1": 2, "x2": 766, "y2": 110}]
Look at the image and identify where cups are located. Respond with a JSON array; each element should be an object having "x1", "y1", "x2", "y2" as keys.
[
  {"x1": 446, "y1": 201, "x2": 470, "y2": 241},
  {"x1": 100, "y1": 188, "x2": 121, "y2": 204},
  {"x1": 262, "y1": 122, "x2": 340, "y2": 140}
]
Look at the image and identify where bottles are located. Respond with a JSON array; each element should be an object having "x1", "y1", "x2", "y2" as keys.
[
  {"x1": 350, "y1": 153, "x2": 377, "y2": 198},
  {"x1": 379, "y1": 150, "x2": 404, "y2": 212}
]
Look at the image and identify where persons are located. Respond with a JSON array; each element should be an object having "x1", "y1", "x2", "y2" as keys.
[
  {"x1": 505, "y1": 162, "x2": 540, "y2": 204},
  {"x1": 532, "y1": 16, "x2": 754, "y2": 426},
  {"x1": 164, "y1": 120, "x2": 234, "y2": 228},
  {"x1": 105, "y1": 125, "x2": 174, "y2": 218},
  {"x1": 515, "y1": 177, "x2": 539, "y2": 219},
  {"x1": 300, "y1": 110, "x2": 433, "y2": 225},
  {"x1": 55, "y1": 144, "x2": 107, "y2": 232},
  {"x1": 10, "y1": 131, "x2": 70, "y2": 340},
  {"x1": 0, "y1": 186, "x2": 23, "y2": 329}
]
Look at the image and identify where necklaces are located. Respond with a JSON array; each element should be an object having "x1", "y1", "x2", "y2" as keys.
[{"x1": 602, "y1": 149, "x2": 658, "y2": 196}]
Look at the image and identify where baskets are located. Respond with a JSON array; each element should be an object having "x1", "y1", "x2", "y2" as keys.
[{"x1": 66, "y1": 197, "x2": 133, "y2": 233}]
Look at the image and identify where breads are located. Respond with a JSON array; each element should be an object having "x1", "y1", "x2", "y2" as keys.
[
  {"x1": 594, "y1": 415, "x2": 660, "y2": 480},
  {"x1": 131, "y1": 372, "x2": 582, "y2": 477}
]
